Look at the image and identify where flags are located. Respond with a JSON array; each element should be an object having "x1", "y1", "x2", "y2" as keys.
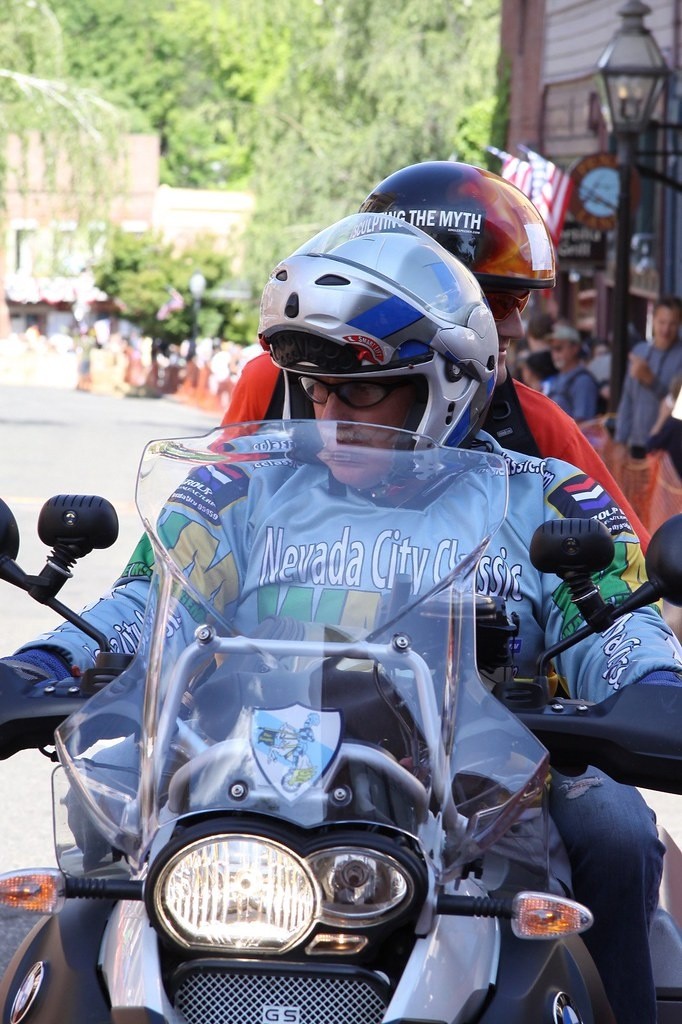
[{"x1": 496, "y1": 144, "x2": 570, "y2": 245}]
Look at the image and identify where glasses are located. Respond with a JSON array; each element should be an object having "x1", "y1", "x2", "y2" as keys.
[
  {"x1": 298, "y1": 377, "x2": 413, "y2": 409},
  {"x1": 549, "y1": 343, "x2": 564, "y2": 352},
  {"x1": 482, "y1": 289, "x2": 532, "y2": 321}
]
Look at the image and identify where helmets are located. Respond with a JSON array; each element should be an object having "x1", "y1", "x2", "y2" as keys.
[
  {"x1": 348, "y1": 161, "x2": 556, "y2": 289},
  {"x1": 257, "y1": 232, "x2": 499, "y2": 481}
]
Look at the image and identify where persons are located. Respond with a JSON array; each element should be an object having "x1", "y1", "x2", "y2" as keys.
[
  {"x1": 224, "y1": 162, "x2": 662, "y2": 693},
  {"x1": 0, "y1": 212, "x2": 682, "y2": 1023},
  {"x1": 19, "y1": 293, "x2": 682, "y2": 637}
]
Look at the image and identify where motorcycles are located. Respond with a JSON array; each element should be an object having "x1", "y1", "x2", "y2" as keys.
[{"x1": 2, "y1": 418, "x2": 682, "y2": 1024}]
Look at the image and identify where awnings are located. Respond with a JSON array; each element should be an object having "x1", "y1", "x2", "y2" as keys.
[{"x1": 7, "y1": 270, "x2": 108, "y2": 308}]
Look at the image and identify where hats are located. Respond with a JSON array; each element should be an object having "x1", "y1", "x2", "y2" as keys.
[{"x1": 546, "y1": 327, "x2": 581, "y2": 344}]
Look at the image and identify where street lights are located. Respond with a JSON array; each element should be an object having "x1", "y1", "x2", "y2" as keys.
[{"x1": 595, "y1": 0, "x2": 675, "y2": 435}]
